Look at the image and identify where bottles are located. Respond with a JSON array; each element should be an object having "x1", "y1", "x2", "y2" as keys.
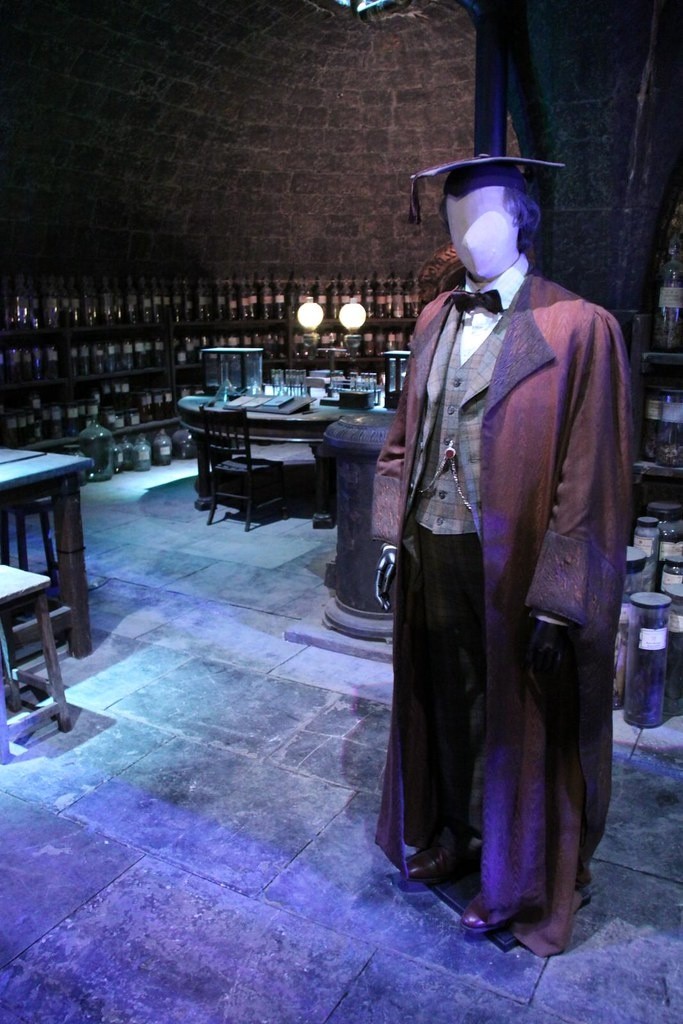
[
  {"x1": 214, "y1": 270, "x2": 418, "y2": 400},
  {"x1": 651, "y1": 257, "x2": 683, "y2": 354},
  {"x1": 0, "y1": 266, "x2": 213, "y2": 481},
  {"x1": 634, "y1": 517, "x2": 660, "y2": 592}
]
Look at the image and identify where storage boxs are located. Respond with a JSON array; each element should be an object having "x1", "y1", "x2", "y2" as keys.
[
  {"x1": 201, "y1": 346, "x2": 265, "y2": 395},
  {"x1": 338, "y1": 391, "x2": 374, "y2": 410},
  {"x1": 385, "y1": 350, "x2": 411, "y2": 408}
]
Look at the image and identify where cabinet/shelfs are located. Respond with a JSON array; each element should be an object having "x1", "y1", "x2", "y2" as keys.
[
  {"x1": 626, "y1": 313, "x2": 683, "y2": 544},
  {"x1": 0, "y1": 309, "x2": 417, "y2": 459}
]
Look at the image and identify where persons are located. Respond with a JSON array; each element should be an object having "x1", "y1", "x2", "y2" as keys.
[{"x1": 373, "y1": 149, "x2": 648, "y2": 956}]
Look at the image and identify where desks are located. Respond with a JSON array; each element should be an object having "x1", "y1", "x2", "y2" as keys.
[
  {"x1": 177, "y1": 387, "x2": 388, "y2": 531},
  {"x1": 0, "y1": 447, "x2": 96, "y2": 661}
]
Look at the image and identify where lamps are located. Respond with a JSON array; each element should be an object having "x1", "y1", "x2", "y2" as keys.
[
  {"x1": 339, "y1": 304, "x2": 367, "y2": 358},
  {"x1": 298, "y1": 303, "x2": 324, "y2": 363}
]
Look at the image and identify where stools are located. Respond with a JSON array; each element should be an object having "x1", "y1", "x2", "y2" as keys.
[{"x1": 0, "y1": 564, "x2": 71, "y2": 764}]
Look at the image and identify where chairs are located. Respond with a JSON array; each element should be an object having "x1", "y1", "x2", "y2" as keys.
[{"x1": 199, "y1": 404, "x2": 289, "y2": 532}]
